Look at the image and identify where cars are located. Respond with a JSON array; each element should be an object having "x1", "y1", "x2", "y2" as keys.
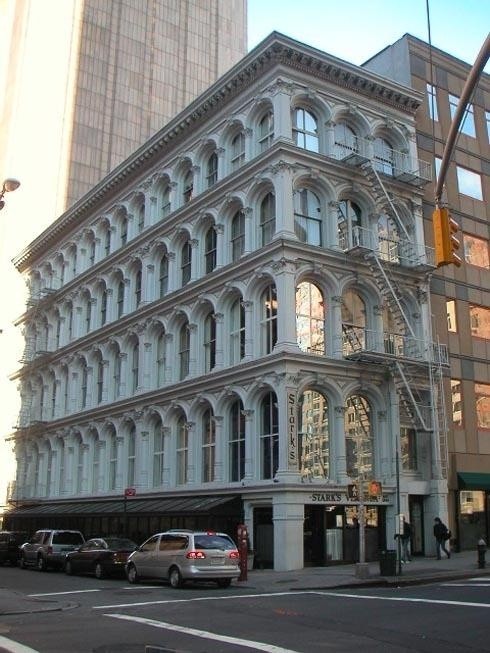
[{"x1": 63, "y1": 538, "x2": 139, "y2": 579}]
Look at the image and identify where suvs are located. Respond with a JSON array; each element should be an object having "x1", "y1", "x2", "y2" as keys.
[
  {"x1": 0, "y1": 531, "x2": 29, "y2": 566},
  {"x1": 18, "y1": 529, "x2": 85, "y2": 571}
]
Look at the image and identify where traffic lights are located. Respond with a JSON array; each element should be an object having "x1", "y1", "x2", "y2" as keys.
[
  {"x1": 434, "y1": 209, "x2": 463, "y2": 269},
  {"x1": 368, "y1": 482, "x2": 382, "y2": 497},
  {"x1": 347, "y1": 484, "x2": 358, "y2": 497}
]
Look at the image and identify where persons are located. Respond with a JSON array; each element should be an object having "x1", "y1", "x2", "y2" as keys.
[
  {"x1": 400, "y1": 521, "x2": 412, "y2": 564},
  {"x1": 433, "y1": 517, "x2": 452, "y2": 560}
]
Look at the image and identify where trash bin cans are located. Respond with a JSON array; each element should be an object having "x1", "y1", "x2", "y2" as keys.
[{"x1": 378, "y1": 549, "x2": 397, "y2": 577}]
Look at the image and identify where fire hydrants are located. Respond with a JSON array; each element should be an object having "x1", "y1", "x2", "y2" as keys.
[{"x1": 477, "y1": 539, "x2": 487, "y2": 567}]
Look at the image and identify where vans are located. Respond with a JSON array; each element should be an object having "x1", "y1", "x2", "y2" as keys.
[{"x1": 124, "y1": 531, "x2": 241, "y2": 589}]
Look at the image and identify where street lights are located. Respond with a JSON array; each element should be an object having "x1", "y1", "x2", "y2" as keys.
[{"x1": 0, "y1": 178, "x2": 20, "y2": 208}]
